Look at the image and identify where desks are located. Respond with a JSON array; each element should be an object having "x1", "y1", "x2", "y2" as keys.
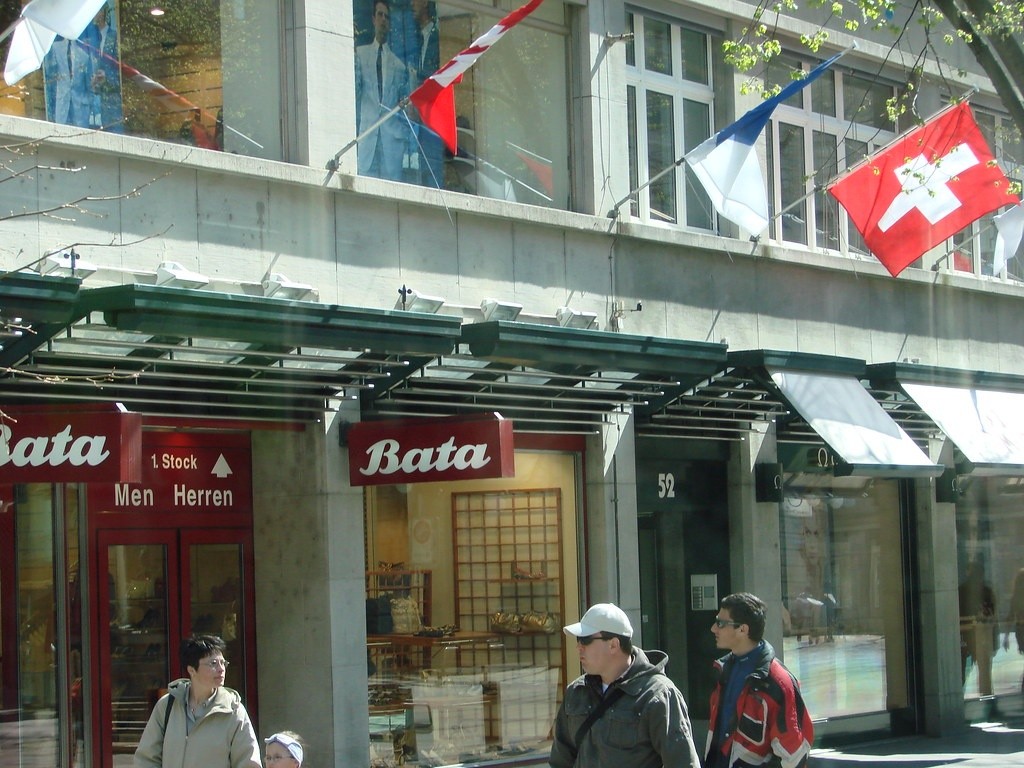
[{"x1": 366, "y1": 630, "x2": 498, "y2": 641}]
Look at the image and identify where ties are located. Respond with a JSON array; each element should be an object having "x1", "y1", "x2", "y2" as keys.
[
  {"x1": 376, "y1": 47, "x2": 383, "y2": 103},
  {"x1": 67, "y1": 39, "x2": 73, "y2": 80}
]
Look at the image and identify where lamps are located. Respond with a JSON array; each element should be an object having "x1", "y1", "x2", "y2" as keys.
[
  {"x1": 262, "y1": 279, "x2": 312, "y2": 300},
  {"x1": 156, "y1": 258, "x2": 210, "y2": 291},
  {"x1": 479, "y1": 299, "x2": 523, "y2": 321},
  {"x1": 936, "y1": 468, "x2": 959, "y2": 505},
  {"x1": 399, "y1": 288, "x2": 445, "y2": 312},
  {"x1": 557, "y1": 306, "x2": 594, "y2": 332},
  {"x1": 757, "y1": 461, "x2": 786, "y2": 503},
  {"x1": 29, "y1": 247, "x2": 96, "y2": 280}
]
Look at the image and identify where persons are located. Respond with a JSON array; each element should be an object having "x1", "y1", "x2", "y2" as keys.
[
  {"x1": 355, "y1": 0, "x2": 444, "y2": 189},
  {"x1": 958, "y1": 562, "x2": 1007, "y2": 722},
  {"x1": 134, "y1": 635, "x2": 263, "y2": 768},
  {"x1": 1003, "y1": 568, "x2": 1024, "y2": 693},
  {"x1": 44, "y1": 1, "x2": 123, "y2": 134},
  {"x1": 264, "y1": 731, "x2": 304, "y2": 768},
  {"x1": 791, "y1": 505, "x2": 835, "y2": 645},
  {"x1": 548, "y1": 602, "x2": 701, "y2": 768},
  {"x1": 700, "y1": 592, "x2": 815, "y2": 768}
]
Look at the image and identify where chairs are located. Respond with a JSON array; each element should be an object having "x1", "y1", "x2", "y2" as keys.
[{"x1": 369, "y1": 673, "x2": 557, "y2": 763}]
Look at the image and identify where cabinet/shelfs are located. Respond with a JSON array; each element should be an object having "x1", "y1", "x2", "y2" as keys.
[
  {"x1": 453, "y1": 487, "x2": 567, "y2": 699},
  {"x1": 368, "y1": 569, "x2": 432, "y2": 675}
]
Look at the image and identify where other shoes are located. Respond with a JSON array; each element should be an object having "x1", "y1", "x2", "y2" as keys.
[
  {"x1": 511, "y1": 560, "x2": 548, "y2": 580},
  {"x1": 106, "y1": 603, "x2": 167, "y2": 731},
  {"x1": 190, "y1": 614, "x2": 214, "y2": 633}
]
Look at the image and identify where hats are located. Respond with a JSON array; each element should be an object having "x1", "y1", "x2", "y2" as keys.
[
  {"x1": 264, "y1": 731, "x2": 303, "y2": 768},
  {"x1": 562, "y1": 603, "x2": 633, "y2": 641}
]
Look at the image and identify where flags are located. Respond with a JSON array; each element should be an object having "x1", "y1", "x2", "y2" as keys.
[
  {"x1": 409, "y1": 0, "x2": 544, "y2": 155},
  {"x1": 79, "y1": 43, "x2": 221, "y2": 151},
  {"x1": 827, "y1": 100, "x2": 1022, "y2": 279},
  {"x1": 3, "y1": 0, "x2": 107, "y2": 87},
  {"x1": 683, "y1": 53, "x2": 843, "y2": 238}
]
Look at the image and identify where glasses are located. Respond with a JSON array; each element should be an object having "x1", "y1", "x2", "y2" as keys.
[
  {"x1": 376, "y1": 10, "x2": 389, "y2": 21},
  {"x1": 199, "y1": 658, "x2": 229, "y2": 671},
  {"x1": 715, "y1": 616, "x2": 752, "y2": 632},
  {"x1": 263, "y1": 753, "x2": 294, "y2": 763},
  {"x1": 577, "y1": 634, "x2": 622, "y2": 646}
]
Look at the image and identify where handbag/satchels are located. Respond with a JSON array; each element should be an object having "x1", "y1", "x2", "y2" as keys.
[
  {"x1": 376, "y1": 561, "x2": 412, "y2": 599},
  {"x1": 220, "y1": 598, "x2": 239, "y2": 642},
  {"x1": 124, "y1": 570, "x2": 156, "y2": 599},
  {"x1": 389, "y1": 590, "x2": 421, "y2": 634},
  {"x1": 519, "y1": 609, "x2": 559, "y2": 634},
  {"x1": 366, "y1": 585, "x2": 394, "y2": 636},
  {"x1": 491, "y1": 609, "x2": 520, "y2": 634}
]
